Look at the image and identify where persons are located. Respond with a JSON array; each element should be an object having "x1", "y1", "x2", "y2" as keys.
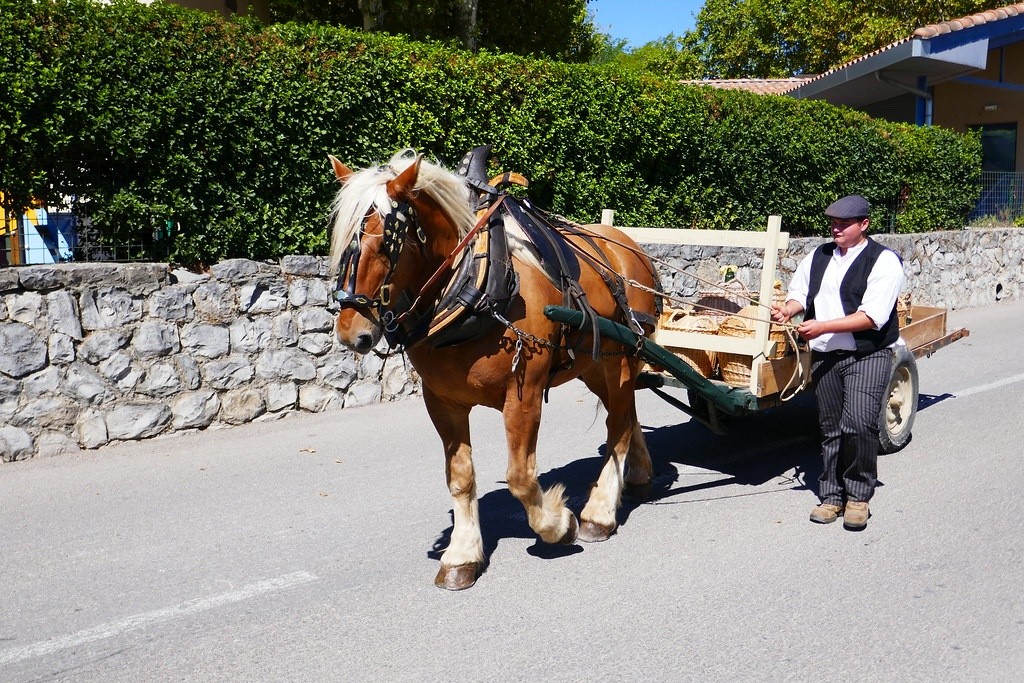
[{"x1": 770, "y1": 195, "x2": 905, "y2": 529}]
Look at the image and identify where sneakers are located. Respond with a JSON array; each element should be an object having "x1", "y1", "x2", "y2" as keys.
[
  {"x1": 810, "y1": 502, "x2": 844, "y2": 524},
  {"x1": 844, "y1": 500, "x2": 870, "y2": 529}
]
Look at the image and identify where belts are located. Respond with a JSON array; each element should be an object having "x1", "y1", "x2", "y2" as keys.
[{"x1": 836, "y1": 348, "x2": 847, "y2": 355}]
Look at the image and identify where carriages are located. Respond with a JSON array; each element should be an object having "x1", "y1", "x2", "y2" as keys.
[{"x1": 326, "y1": 143, "x2": 969, "y2": 593}]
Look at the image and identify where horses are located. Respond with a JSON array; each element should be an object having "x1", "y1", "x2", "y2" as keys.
[{"x1": 327, "y1": 148, "x2": 662, "y2": 591}]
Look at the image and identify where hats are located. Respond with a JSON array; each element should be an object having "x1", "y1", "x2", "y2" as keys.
[{"x1": 824, "y1": 195, "x2": 869, "y2": 220}]
